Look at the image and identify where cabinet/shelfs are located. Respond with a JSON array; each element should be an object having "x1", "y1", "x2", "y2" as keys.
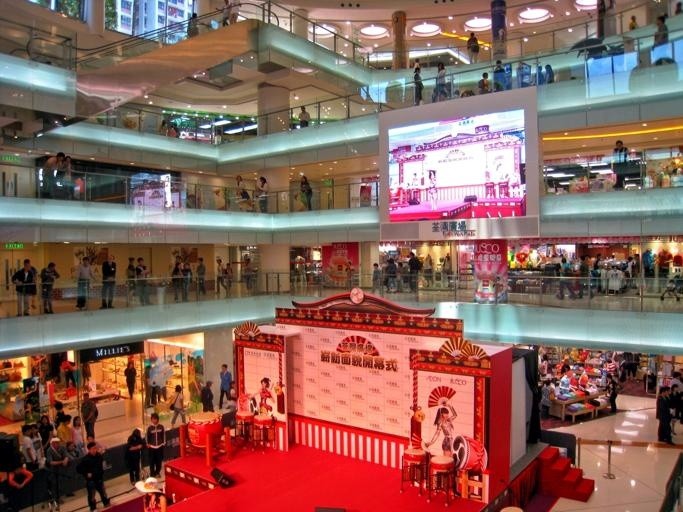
[{"x1": 100, "y1": 352, "x2": 186, "y2": 403}]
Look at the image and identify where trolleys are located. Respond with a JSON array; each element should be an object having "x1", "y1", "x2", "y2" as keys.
[{"x1": 660, "y1": 275, "x2": 683, "y2": 301}]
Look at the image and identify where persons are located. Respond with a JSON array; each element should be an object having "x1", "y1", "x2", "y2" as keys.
[
  {"x1": 230, "y1": 1, "x2": 241, "y2": 25},
  {"x1": 244, "y1": 258, "x2": 251, "y2": 292},
  {"x1": 423, "y1": 253, "x2": 434, "y2": 287},
  {"x1": 144, "y1": 360, "x2": 167, "y2": 408},
  {"x1": 235, "y1": 175, "x2": 249, "y2": 200},
  {"x1": 81, "y1": 362, "x2": 90, "y2": 386},
  {"x1": 432, "y1": 88, "x2": 436, "y2": 101},
  {"x1": 633, "y1": 253, "x2": 641, "y2": 295},
  {"x1": 675, "y1": 3, "x2": 681, "y2": 13},
  {"x1": 300, "y1": 175, "x2": 311, "y2": 210},
  {"x1": 219, "y1": 364, "x2": 231, "y2": 409},
  {"x1": 539, "y1": 353, "x2": 644, "y2": 427},
  {"x1": 494, "y1": 61, "x2": 505, "y2": 89},
  {"x1": 384, "y1": 252, "x2": 418, "y2": 293},
  {"x1": 169, "y1": 385, "x2": 185, "y2": 428},
  {"x1": 256, "y1": 176, "x2": 267, "y2": 213},
  {"x1": 612, "y1": 141, "x2": 629, "y2": 189},
  {"x1": 654, "y1": 17, "x2": 668, "y2": 46},
  {"x1": 158, "y1": 120, "x2": 179, "y2": 138},
  {"x1": 126, "y1": 429, "x2": 144, "y2": 484},
  {"x1": 436, "y1": 63, "x2": 446, "y2": 100},
  {"x1": 215, "y1": 1, "x2": 229, "y2": 26},
  {"x1": 594, "y1": 254, "x2": 600, "y2": 269},
  {"x1": 229, "y1": 381, "x2": 234, "y2": 397},
  {"x1": 21, "y1": 393, "x2": 110, "y2": 511},
  {"x1": 577, "y1": 256, "x2": 594, "y2": 299},
  {"x1": 42, "y1": 152, "x2": 70, "y2": 198},
  {"x1": 371, "y1": 263, "x2": 383, "y2": 297},
  {"x1": 200, "y1": 380, "x2": 213, "y2": 412},
  {"x1": 479, "y1": 73, "x2": 488, "y2": 93},
  {"x1": 535, "y1": 66, "x2": 544, "y2": 84},
  {"x1": 12, "y1": 254, "x2": 233, "y2": 316},
  {"x1": 60, "y1": 357, "x2": 76, "y2": 387},
  {"x1": 258, "y1": 378, "x2": 271, "y2": 413},
  {"x1": 124, "y1": 362, "x2": 136, "y2": 398},
  {"x1": 467, "y1": 33, "x2": 479, "y2": 64},
  {"x1": 413, "y1": 59, "x2": 419, "y2": 68},
  {"x1": 298, "y1": 106, "x2": 309, "y2": 127},
  {"x1": 426, "y1": 404, "x2": 457, "y2": 457},
  {"x1": 455, "y1": 90, "x2": 460, "y2": 97},
  {"x1": 414, "y1": 68, "x2": 424, "y2": 105},
  {"x1": 545, "y1": 66, "x2": 554, "y2": 83},
  {"x1": 655, "y1": 373, "x2": 683, "y2": 443},
  {"x1": 189, "y1": 14, "x2": 199, "y2": 36},
  {"x1": 344, "y1": 262, "x2": 352, "y2": 293},
  {"x1": 144, "y1": 413, "x2": 165, "y2": 477},
  {"x1": 517, "y1": 62, "x2": 524, "y2": 86},
  {"x1": 556, "y1": 258, "x2": 576, "y2": 300},
  {"x1": 629, "y1": 16, "x2": 636, "y2": 30}
]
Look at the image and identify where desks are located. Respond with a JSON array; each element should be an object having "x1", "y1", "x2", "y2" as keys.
[{"x1": 547, "y1": 386, "x2": 613, "y2": 423}]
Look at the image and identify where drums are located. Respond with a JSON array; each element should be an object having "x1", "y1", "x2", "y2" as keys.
[
  {"x1": 187, "y1": 412, "x2": 221, "y2": 449},
  {"x1": 251, "y1": 414, "x2": 274, "y2": 428},
  {"x1": 450, "y1": 436, "x2": 489, "y2": 471},
  {"x1": 429, "y1": 456, "x2": 454, "y2": 473},
  {"x1": 235, "y1": 412, "x2": 253, "y2": 423},
  {"x1": 237, "y1": 393, "x2": 257, "y2": 414},
  {"x1": 404, "y1": 449, "x2": 427, "y2": 464}
]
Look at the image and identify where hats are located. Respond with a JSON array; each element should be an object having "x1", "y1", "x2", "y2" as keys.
[
  {"x1": 135, "y1": 477, "x2": 161, "y2": 493},
  {"x1": 386, "y1": 258, "x2": 394, "y2": 263}
]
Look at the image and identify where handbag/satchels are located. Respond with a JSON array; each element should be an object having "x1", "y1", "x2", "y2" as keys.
[
  {"x1": 29, "y1": 461, "x2": 38, "y2": 469},
  {"x1": 170, "y1": 403, "x2": 175, "y2": 410},
  {"x1": 550, "y1": 390, "x2": 555, "y2": 400}
]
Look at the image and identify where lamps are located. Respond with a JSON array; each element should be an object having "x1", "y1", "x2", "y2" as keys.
[
  {"x1": 408, "y1": 20, "x2": 443, "y2": 38},
  {"x1": 463, "y1": 14, "x2": 491, "y2": 31},
  {"x1": 516, "y1": 4, "x2": 553, "y2": 24},
  {"x1": 306, "y1": 23, "x2": 340, "y2": 39},
  {"x1": 572, "y1": 1, "x2": 597, "y2": 11},
  {"x1": 356, "y1": 22, "x2": 390, "y2": 40}
]
